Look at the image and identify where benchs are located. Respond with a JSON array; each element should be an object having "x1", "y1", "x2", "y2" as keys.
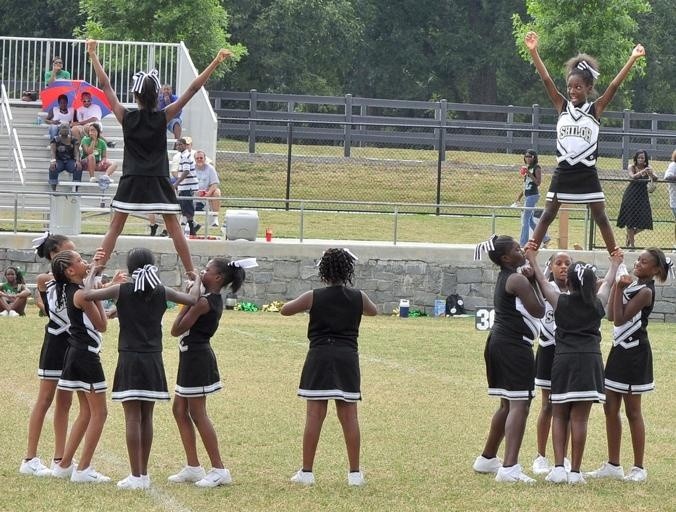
[{"x1": 1, "y1": 97, "x2": 224, "y2": 230}]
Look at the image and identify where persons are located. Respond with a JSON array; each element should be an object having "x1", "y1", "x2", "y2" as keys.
[
  {"x1": 523, "y1": 31, "x2": 645, "y2": 253},
  {"x1": 83, "y1": 247, "x2": 201, "y2": 491},
  {"x1": 586, "y1": 248, "x2": 672, "y2": 482},
  {"x1": 663, "y1": 149, "x2": 676, "y2": 248},
  {"x1": 40, "y1": 58, "x2": 220, "y2": 237},
  {"x1": 473, "y1": 234, "x2": 546, "y2": 483},
  {"x1": 51, "y1": 249, "x2": 111, "y2": 483},
  {"x1": 167, "y1": 257, "x2": 246, "y2": 489},
  {"x1": 0, "y1": 267, "x2": 32, "y2": 318},
  {"x1": 17, "y1": 232, "x2": 127, "y2": 479},
  {"x1": 281, "y1": 246, "x2": 377, "y2": 487},
  {"x1": 84, "y1": 38, "x2": 232, "y2": 286},
  {"x1": 513, "y1": 147, "x2": 551, "y2": 249},
  {"x1": 521, "y1": 238, "x2": 626, "y2": 483},
  {"x1": 617, "y1": 146, "x2": 659, "y2": 249}
]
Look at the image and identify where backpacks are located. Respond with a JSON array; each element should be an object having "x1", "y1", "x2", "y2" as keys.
[{"x1": 445, "y1": 294, "x2": 463, "y2": 315}]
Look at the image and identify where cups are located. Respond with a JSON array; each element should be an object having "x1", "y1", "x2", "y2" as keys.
[
  {"x1": 265, "y1": 227, "x2": 272, "y2": 242},
  {"x1": 400, "y1": 298, "x2": 410, "y2": 317},
  {"x1": 198, "y1": 191, "x2": 205, "y2": 196},
  {"x1": 520, "y1": 166, "x2": 527, "y2": 177}
]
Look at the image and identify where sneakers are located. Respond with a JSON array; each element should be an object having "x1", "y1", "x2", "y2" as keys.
[
  {"x1": 347, "y1": 472, "x2": 362, "y2": 487},
  {"x1": 168, "y1": 465, "x2": 232, "y2": 487},
  {"x1": 19, "y1": 456, "x2": 111, "y2": 483},
  {"x1": 150, "y1": 220, "x2": 201, "y2": 237},
  {"x1": 117, "y1": 474, "x2": 150, "y2": 489},
  {"x1": 615, "y1": 262, "x2": 629, "y2": 286},
  {"x1": 0, "y1": 309, "x2": 20, "y2": 316},
  {"x1": 291, "y1": 468, "x2": 315, "y2": 483},
  {"x1": 516, "y1": 258, "x2": 531, "y2": 273},
  {"x1": 473, "y1": 454, "x2": 646, "y2": 484}
]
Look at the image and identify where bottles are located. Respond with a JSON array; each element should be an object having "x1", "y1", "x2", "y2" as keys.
[
  {"x1": 225, "y1": 292, "x2": 237, "y2": 310},
  {"x1": 37, "y1": 115, "x2": 41, "y2": 124},
  {"x1": 222, "y1": 218, "x2": 227, "y2": 238},
  {"x1": 183, "y1": 222, "x2": 190, "y2": 240}
]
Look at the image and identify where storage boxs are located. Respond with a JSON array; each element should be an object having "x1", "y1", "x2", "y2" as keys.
[{"x1": 224, "y1": 208, "x2": 260, "y2": 241}]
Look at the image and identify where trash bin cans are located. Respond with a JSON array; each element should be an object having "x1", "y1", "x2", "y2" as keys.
[{"x1": 50, "y1": 193, "x2": 80, "y2": 236}]
